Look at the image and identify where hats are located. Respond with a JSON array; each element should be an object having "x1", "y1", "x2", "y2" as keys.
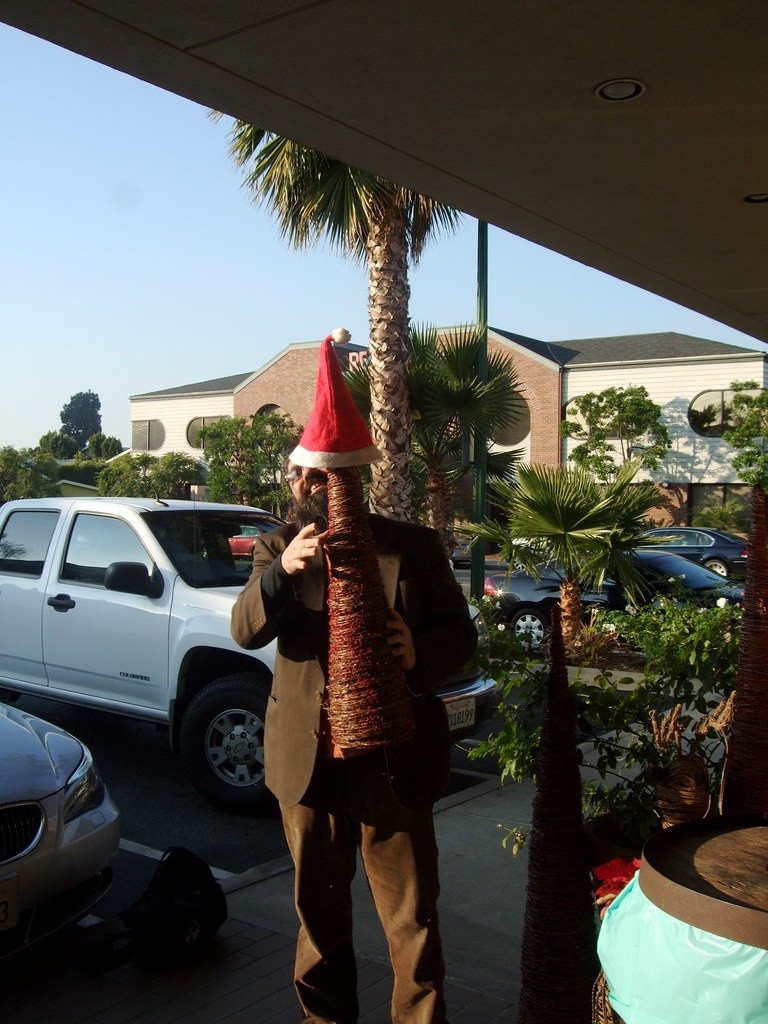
[{"x1": 289, "y1": 327, "x2": 384, "y2": 468}]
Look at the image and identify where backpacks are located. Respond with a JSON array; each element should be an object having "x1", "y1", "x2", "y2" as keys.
[{"x1": 96, "y1": 845, "x2": 227, "y2": 972}]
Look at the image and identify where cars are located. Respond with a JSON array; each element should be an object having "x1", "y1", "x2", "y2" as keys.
[
  {"x1": 445, "y1": 531, "x2": 550, "y2": 573},
  {"x1": 622, "y1": 524, "x2": 748, "y2": 582},
  {"x1": 483, "y1": 547, "x2": 745, "y2": 659},
  {"x1": 0, "y1": 699, "x2": 124, "y2": 965}
]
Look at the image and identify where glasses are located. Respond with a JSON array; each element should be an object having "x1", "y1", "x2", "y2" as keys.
[{"x1": 284, "y1": 465, "x2": 304, "y2": 486}]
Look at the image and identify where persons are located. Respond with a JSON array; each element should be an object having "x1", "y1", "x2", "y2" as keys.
[{"x1": 231, "y1": 445, "x2": 483, "y2": 1022}]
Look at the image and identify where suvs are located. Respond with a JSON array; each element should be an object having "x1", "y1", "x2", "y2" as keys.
[{"x1": 0, "y1": 494, "x2": 506, "y2": 821}]
